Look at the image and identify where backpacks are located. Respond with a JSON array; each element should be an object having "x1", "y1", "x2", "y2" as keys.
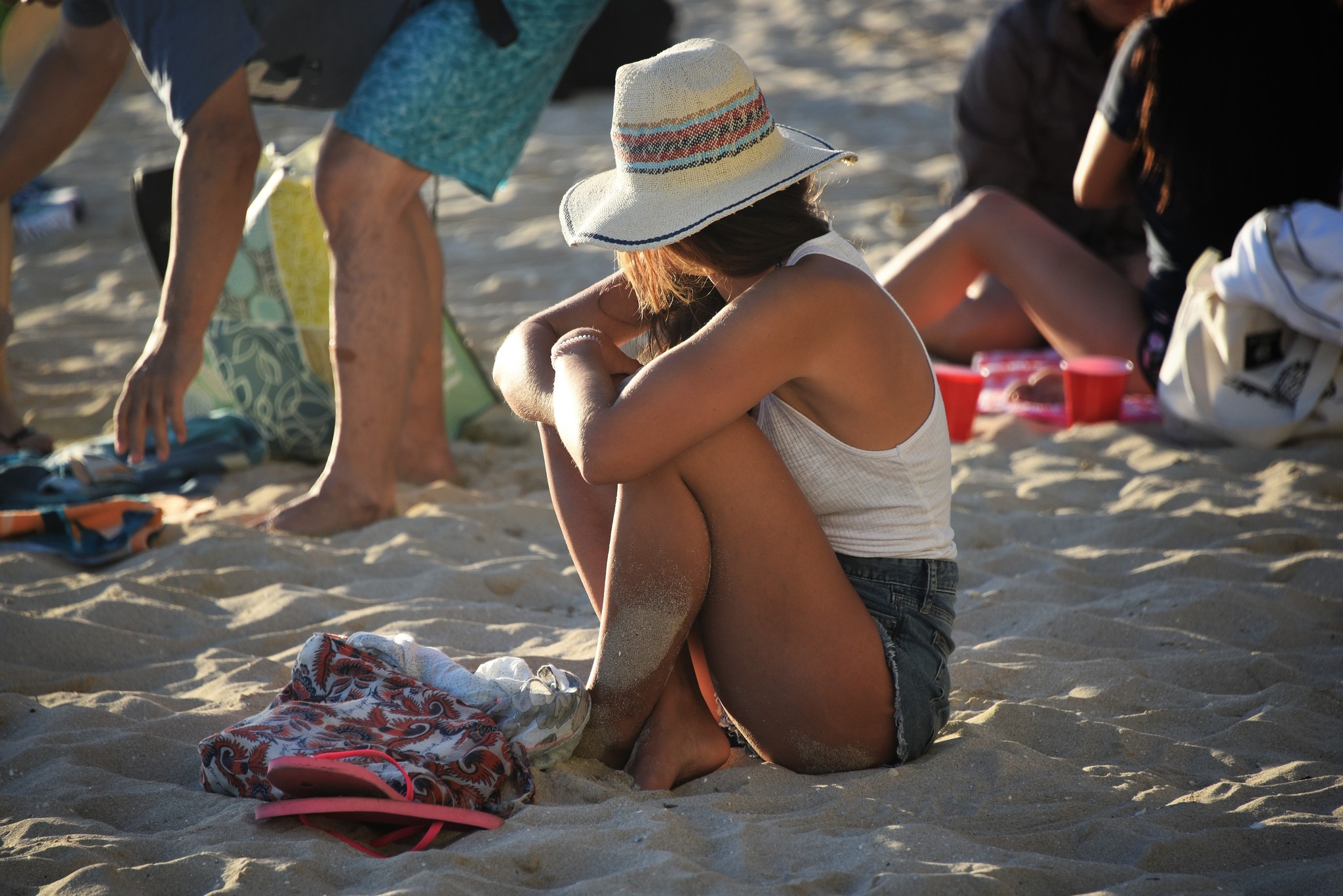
[{"x1": 1144, "y1": 193, "x2": 1343, "y2": 443}]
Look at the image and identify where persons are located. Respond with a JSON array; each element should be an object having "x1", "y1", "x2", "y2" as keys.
[
  {"x1": 489, "y1": 38, "x2": 956, "y2": 788},
  {"x1": 926, "y1": 1, "x2": 1164, "y2": 364},
  {"x1": 1, "y1": 0, "x2": 617, "y2": 536},
  {"x1": 873, "y1": 0, "x2": 1343, "y2": 401}
]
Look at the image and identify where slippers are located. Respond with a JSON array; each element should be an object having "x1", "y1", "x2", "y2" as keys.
[
  {"x1": 262, "y1": 749, "x2": 413, "y2": 797},
  {"x1": 253, "y1": 797, "x2": 508, "y2": 861}
]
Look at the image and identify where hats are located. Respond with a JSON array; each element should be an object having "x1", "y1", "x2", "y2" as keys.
[{"x1": 559, "y1": 30, "x2": 859, "y2": 249}]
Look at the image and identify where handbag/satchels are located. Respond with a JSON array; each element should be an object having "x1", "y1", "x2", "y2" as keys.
[{"x1": 192, "y1": 129, "x2": 502, "y2": 464}]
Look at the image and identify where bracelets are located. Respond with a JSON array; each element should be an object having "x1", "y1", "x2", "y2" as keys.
[{"x1": 550, "y1": 335, "x2": 604, "y2": 371}]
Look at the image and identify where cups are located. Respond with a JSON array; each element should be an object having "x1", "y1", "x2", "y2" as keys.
[
  {"x1": 1059, "y1": 355, "x2": 1133, "y2": 430},
  {"x1": 935, "y1": 365, "x2": 990, "y2": 441}
]
